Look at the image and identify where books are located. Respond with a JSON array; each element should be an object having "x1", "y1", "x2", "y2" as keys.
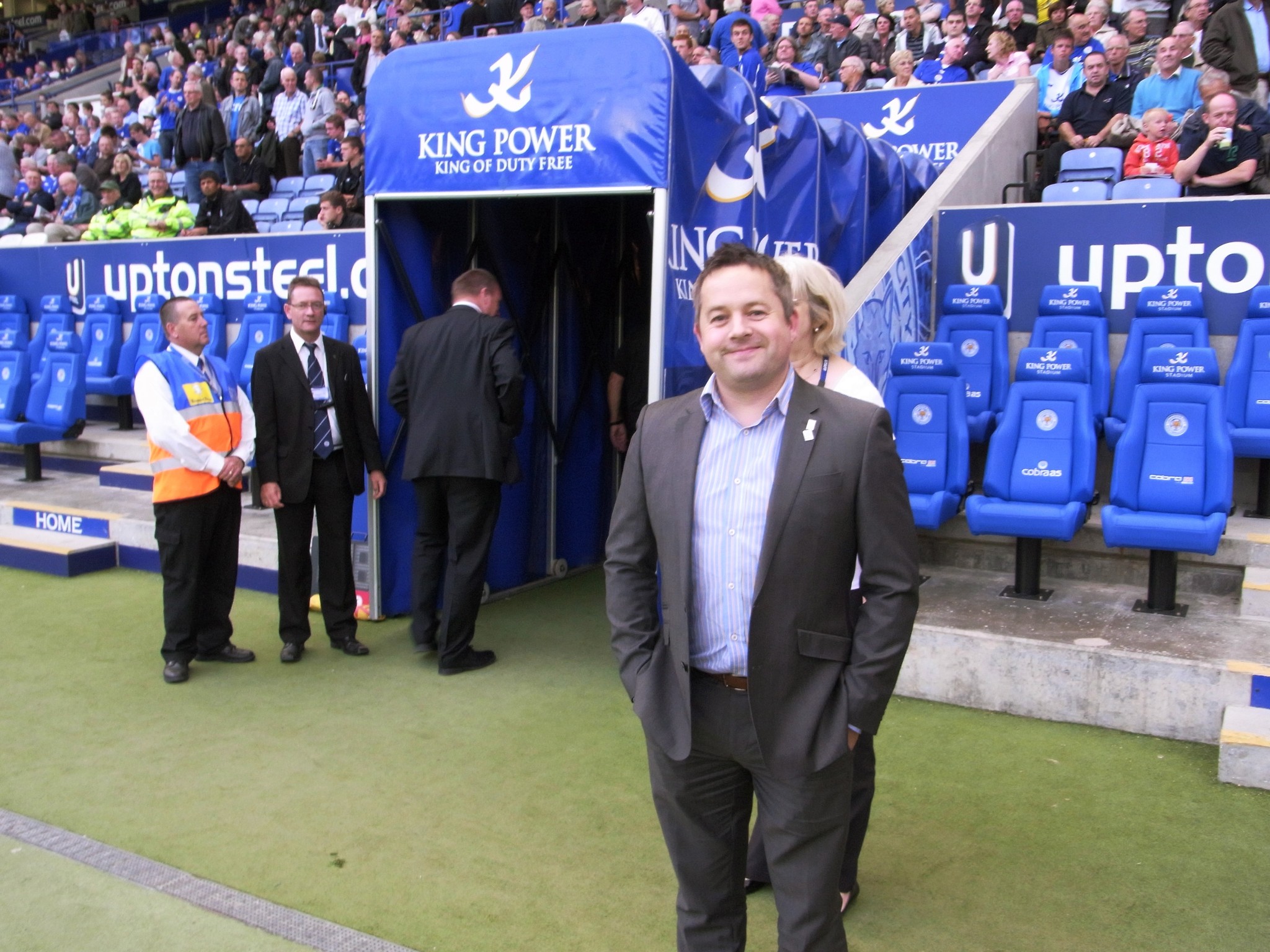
[
  {"x1": 766, "y1": 65, "x2": 799, "y2": 86},
  {"x1": 818, "y1": 66, "x2": 825, "y2": 82}
]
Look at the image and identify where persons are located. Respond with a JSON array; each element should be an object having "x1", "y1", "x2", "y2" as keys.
[
  {"x1": 603, "y1": 241, "x2": 921, "y2": 952},
  {"x1": 605, "y1": 316, "x2": 650, "y2": 484},
  {"x1": 0, "y1": 0, "x2": 1270, "y2": 243},
  {"x1": 743, "y1": 254, "x2": 890, "y2": 923},
  {"x1": 251, "y1": 277, "x2": 388, "y2": 665},
  {"x1": 133, "y1": 296, "x2": 257, "y2": 683},
  {"x1": 389, "y1": 269, "x2": 526, "y2": 677}
]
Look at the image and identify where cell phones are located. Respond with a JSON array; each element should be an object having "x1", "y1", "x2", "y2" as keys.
[{"x1": 317, "y1": 159, "x2": 322, "y2": 162}]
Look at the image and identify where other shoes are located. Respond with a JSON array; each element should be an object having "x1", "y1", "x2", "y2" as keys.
[
  {"x1": 438, "y1": 649, "x2": 496, "y2": 674},
  {"x1": 415, "y1": 642, "x2": 437, "y2": 653}
]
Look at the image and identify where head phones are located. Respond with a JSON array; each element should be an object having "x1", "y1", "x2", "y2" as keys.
[{"x1": 102, "y1": 197, "x2": 126, "y2": 213}]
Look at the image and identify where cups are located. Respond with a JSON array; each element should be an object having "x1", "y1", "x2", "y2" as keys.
[{"x1": 1218, "y1": 128, "x2": 1233, "y2": 150}]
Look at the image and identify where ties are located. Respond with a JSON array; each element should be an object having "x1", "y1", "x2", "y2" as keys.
[
  {"x1": 302, "y1": 343, "x2": 334, "y2": 461},
  {"x1": 317, "y1": 26, "x2": 323, "y2": 47}
]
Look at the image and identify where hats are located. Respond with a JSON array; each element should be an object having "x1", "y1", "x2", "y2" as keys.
[
  {"x1": 98, "y1": 179, "x2": 119, "y2": 190},
  {"x1": 826, "y1": 14, "x2": 851, "y2": 27}
]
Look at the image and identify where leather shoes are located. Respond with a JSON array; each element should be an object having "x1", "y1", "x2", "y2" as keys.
[
  {"x1": 195, "y1": 644, "x2": 256, "y2": 663},
  {"x1": 163, "y1": 659, "x2": 189, "y2": 683},
  {"x1": 331, "y1": 636, "x2": 370, "y2": 656},
  {"x1": 743, "y1": 879, "x2": 769, "y2": 895},
  {"x1": 280, "y1": 642, "x2": 305, "y2": 664},
  {"x1": 837, "y1": 879, "x2": 860, "y2": 917}
]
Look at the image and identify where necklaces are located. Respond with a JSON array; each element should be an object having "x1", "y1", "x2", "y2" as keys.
[{"x1": 804, "y1": 359, "x2": 822, "y2": 380}]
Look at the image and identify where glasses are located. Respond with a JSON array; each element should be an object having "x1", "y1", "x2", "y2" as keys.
[
  {"x1": 840, "y1": 65, "x2": 853, "y2": 70},
  {"x1": 289, "y1": 301, "x2": 325, "y2": 310},
  {"x1": 151, "y1": 179, "x2": 166, "y2": 182},
  {"x1": 1172, "y1": 34, "x2": 1187, "y2": 38},
  {"x1": 1187, "y1": 2, "x2": 1209, "y2": 9},
  {"x1": 777, "y1": 45, "x2": 793, "y2": 49}
]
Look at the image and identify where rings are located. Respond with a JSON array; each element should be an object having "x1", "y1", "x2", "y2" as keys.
[
  {"x1": 265, "y1": 501, "x2": 268, "y2": 504},
  {"x1": 229, "y1": 471, "x2": 234, "y2": 475}
]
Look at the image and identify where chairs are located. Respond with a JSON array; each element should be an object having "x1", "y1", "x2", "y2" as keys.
[
  {"x1": 141, "y1": 169, "x2": 338, "y2": 232},
  {"x1": 1040, "y1": 147, "x2": 1188, "y2": 203},
  {"x1": 322, "y1": 66, "x2": 356, "y2": 97},
  {"x1": 811, "y1": 78, "x2": 886, "y2": 95},
  {"x1": 0, "y1": 295, "x2": 366, "y2": 490},
  {"x1": 885, "y1": 283, "x2": 1270, "y2": 618}
]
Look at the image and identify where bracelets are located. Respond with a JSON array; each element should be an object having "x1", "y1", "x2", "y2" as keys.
[
  {"x1": 608, "y1": 418, "x2": 624, "y2": 427},
  {"x1": 233, "y1": 185, "x2": 237, "y2": 193}
]
